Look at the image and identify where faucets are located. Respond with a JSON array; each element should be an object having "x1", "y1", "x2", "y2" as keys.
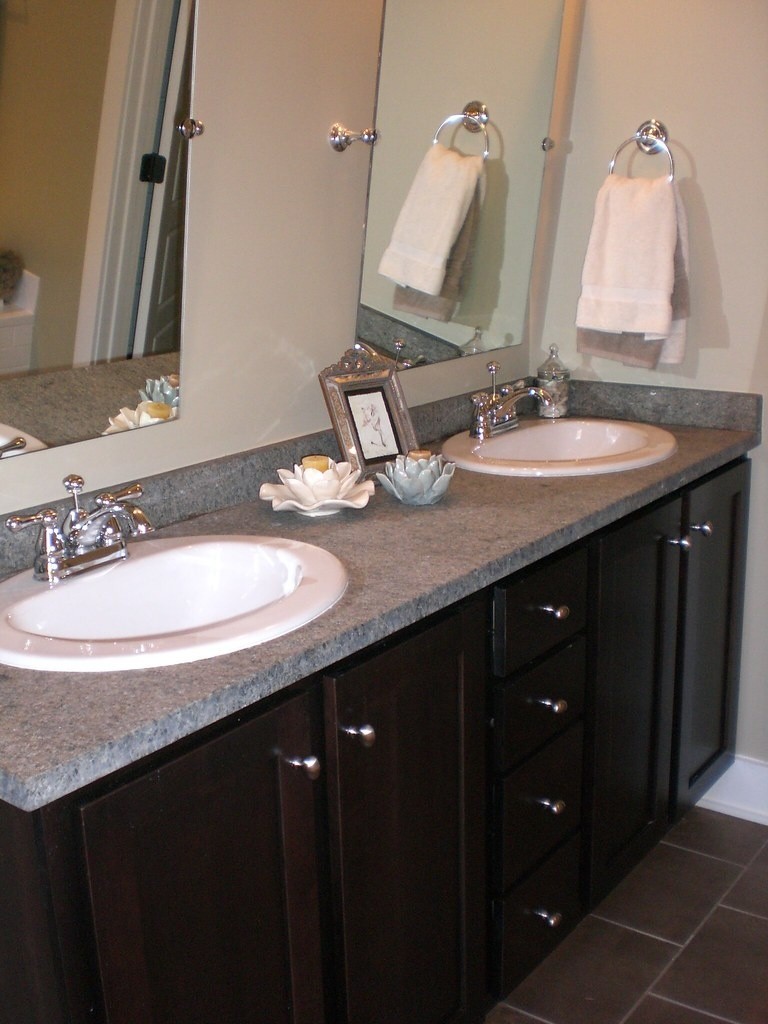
[
  {"x1": 488, "y1": 386, "x2": 554, "y2": 425},
  {"x1": 357, "y1": 341, "x2": 385, "y2": 364},
  {"x1": 66, "y1": 502, "x2": 137, "y2": 547}
]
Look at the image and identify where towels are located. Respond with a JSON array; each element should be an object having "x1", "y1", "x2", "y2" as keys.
[
  {"x1": 575, "y1": 175, "x2": 692, "y2": 370},
  {"x1": 376, "y1": 142, "x2": 487, "y2": 323}
]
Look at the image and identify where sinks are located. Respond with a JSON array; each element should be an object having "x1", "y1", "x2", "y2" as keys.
[
  {"x1": 0, "y1": 534, "x2": 349, "y2": 671},
  {"x1": 1, "y1": 423, "x2": 48, "y2": 459},
  {"x1": 377, "y1": 354, "x2": 405, "y2": 370},
  {"x1": 441, "y1": 416, "x2": 680, "y2": 478}
]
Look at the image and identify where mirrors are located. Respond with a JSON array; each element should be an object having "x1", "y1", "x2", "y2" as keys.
[
  {"x1": 353, "y1": 0, "x2": 566, "y2": 373},
  {"x1": 0, "y1": 0, "x2": 197, "y2": 461}
]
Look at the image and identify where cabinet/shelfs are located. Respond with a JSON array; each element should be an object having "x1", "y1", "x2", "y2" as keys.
[{"x1": 70, "y1": 457, "x2": 751, "y2": 1024}]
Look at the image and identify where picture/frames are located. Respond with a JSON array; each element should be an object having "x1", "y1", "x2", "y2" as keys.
[{"x1": 318, "y1": 350, "x2": 419, "y2": 485}]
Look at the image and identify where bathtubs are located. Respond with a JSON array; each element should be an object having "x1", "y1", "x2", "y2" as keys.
[{"x1": 1, "y1": 300, "x2": 36, "y2": 375}]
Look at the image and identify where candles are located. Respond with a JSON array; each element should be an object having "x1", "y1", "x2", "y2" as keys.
[
  {"x1": 145, "y1": 403, "x2": 171, "y2": 419},
  {"x1": 168, "y1": 374, "x2": 179, "y2": 388},
  {"x1": 302, "y1": 456, "x2": 329, "y2": 474},
  {"x1": 409, "y1": 450, "x2": 430, "y2": 462}
]
